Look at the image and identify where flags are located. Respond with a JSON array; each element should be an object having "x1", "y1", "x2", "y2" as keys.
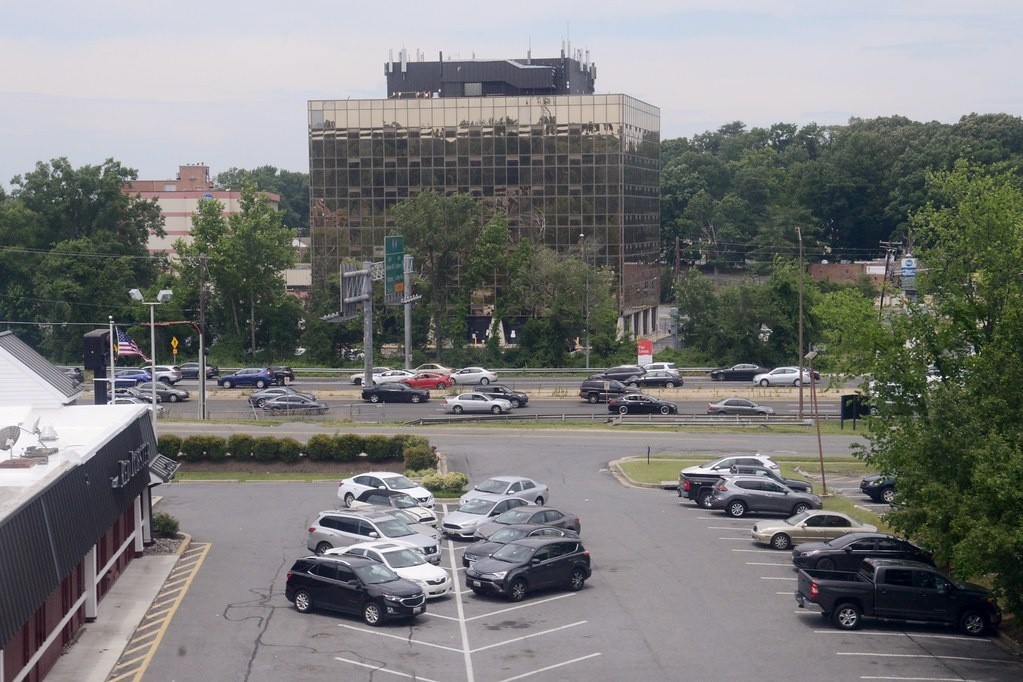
[{"x1": 114, "y1": 325, "x2": 152, "y2": 364}]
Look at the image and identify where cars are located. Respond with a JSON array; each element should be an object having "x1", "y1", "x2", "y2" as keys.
[
  {"x1": 109, "y1": 396, "x2": 164, "y2": 414},
  {"x1": 324, "y1": 542, "x2": 452, "y2": 600},
  {"x1": 860, "y1": 473, "x2": 898, "y2": 504},
  {"x1": 131, "y1": 382, "x2": 190, "y2": 403},
  {"x1": 792, "y1": 533, "x2": 936, "y2": 570},
  {"x1": 461, "y1": 524, "x2": 581, "y2": 569},
  {"x1": 442, "y1": 474, "x2": 582, "y2": 544},
  {"x1": 337, "y1": 469, "x2": 443, "y2": 543},
  {"x1": 465, "y1": 535, "x2": 593, "y2": 602},
  {"x1": 752, "y1": 509, "x2": 879, "y2": 551},
  {"x1": 107, "y1": 369, "x2": 153, "y2": 388},
  {"x1": 142, "y1": 361, "x2": 942, "y2": 415},
  {"x1": 108, "y1": 388, "x2": 162, "y2": 405},
  {"x1": 680, "y1": 454, "x2": 782, "y2": 479}
]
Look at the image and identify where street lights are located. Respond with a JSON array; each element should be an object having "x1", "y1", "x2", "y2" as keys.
[{"x1": 128, "y1": 287, "x2": 175, "y2": 443}]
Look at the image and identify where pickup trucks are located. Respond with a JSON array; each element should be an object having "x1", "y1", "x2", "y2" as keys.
[
  {"x1": 676, "y1": 465, "x2": 814, "y2": 511},
  {"x1": 794, "y1": 559, "x2": 1004, "y2": 636}
]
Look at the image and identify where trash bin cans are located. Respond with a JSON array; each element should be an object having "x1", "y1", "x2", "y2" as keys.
[{"x1": 839, "y1": 394, "x2": 873, "y2": 432}]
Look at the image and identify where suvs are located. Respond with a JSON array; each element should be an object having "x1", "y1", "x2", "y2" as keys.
[
  {"x1": 708, "y1": 475, "x2": 823, "y2": 518},
  {"x1": 303, "y1": 512, "x2": 441, "y2": 564},
  {"x1": 284, "y1": 555, "x2": 428, "y2": 628}
]
